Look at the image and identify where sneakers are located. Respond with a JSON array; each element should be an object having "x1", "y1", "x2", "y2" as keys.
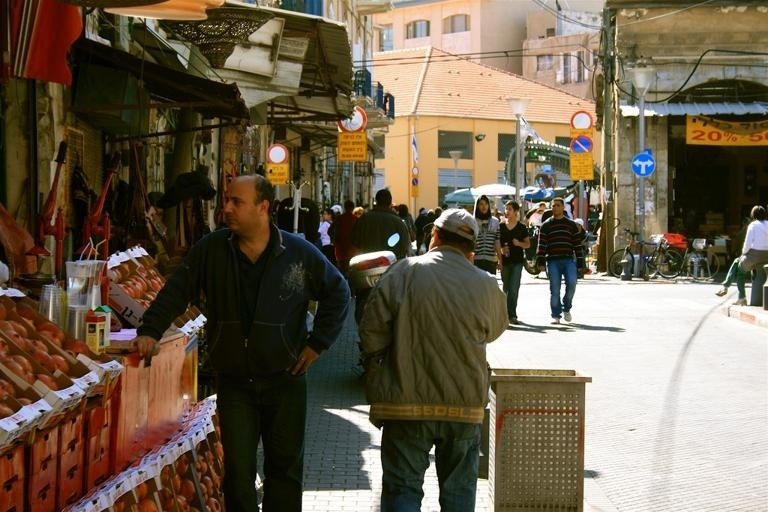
[
  {"x1": 715, "y1": 288, "x2": 727, "y2": 296},
  {"x1": 551, "y1": 317, "x2": 560, "y2": 324},
  {"x1": 564, "y1": 312, "x2": 571, "y2": 321},
  {"x1": 732, "y1": 299, "x2": 747, "y2": 306}
]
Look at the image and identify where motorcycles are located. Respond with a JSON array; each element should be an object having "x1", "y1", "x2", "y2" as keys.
[{"x1": 348, "y1": 232, "x2": 405, "y2": 380}]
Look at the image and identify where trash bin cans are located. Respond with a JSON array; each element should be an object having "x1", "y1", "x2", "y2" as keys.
[{"x1": 489, "y1": 369, "x2": 591, "y2": 511}]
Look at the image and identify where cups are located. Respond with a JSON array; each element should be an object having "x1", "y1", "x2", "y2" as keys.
[{"x1": 39, "y1": 283, "x2": 86, "y2": 341}]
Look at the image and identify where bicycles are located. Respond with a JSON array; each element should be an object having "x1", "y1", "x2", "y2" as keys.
[
  {"x1": 609, "y1": 227, "x2": 684, "y2": 280},
  {"x1": 642, "y1": 238, "x2": 719, "y2": 281}
]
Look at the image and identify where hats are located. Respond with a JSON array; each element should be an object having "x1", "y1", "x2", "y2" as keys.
[{"x1": 433, "y1": 208, "x2": 479, "y2": 242}]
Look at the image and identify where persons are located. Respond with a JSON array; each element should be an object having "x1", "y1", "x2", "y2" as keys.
[
  {"x1": 132, "y1": 174, "x2": 349, "y2": 511},
  {"x1": 715, "y1": 205, "x2": 768, "y2": 305},
  {"x1": 320, "y1": 190, "x2": 450, "y2": 325},
  {"x1": 470, "y1": 196, "x2": 585, "y2": 326},
  {"x1": 726, "y1": 216, "x2": 751, "y2": 261},
  {"x1": 361, "y1": 208, "x2": 510, "y2": 511}
]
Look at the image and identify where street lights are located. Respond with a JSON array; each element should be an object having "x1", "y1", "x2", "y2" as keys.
[
  {"x1": 507, "y1": 96, "x2": 528, "y2": 203},
  {"x1": 448, "y1": 149, "x2": 463, "y2": 189},
  {"x1": 626, "y1": 53, "x2": 661, "y2": 278}
]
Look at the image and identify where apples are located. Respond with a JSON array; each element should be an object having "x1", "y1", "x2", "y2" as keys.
[
  {"x1": 113, "y1": 406, "x2": 225, "y2": 512},
  {"x1": 113, "y1": 264, "x2": 163, "y2": 312},
  {"x1": 0, "y1": 298, "x2": 102, "y2": 416}
]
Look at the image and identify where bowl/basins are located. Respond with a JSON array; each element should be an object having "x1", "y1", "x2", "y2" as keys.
[{"x1": 663, "y1": 232, "x2": 683, "y2": 242}]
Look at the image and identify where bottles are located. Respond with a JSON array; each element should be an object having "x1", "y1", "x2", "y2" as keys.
[
  {"x1": 65, "y1": 258, "x2": 107, "y2": 308},
  {"x1": 503, "y1": 241, "x2": 510, "y2": 258}
]
